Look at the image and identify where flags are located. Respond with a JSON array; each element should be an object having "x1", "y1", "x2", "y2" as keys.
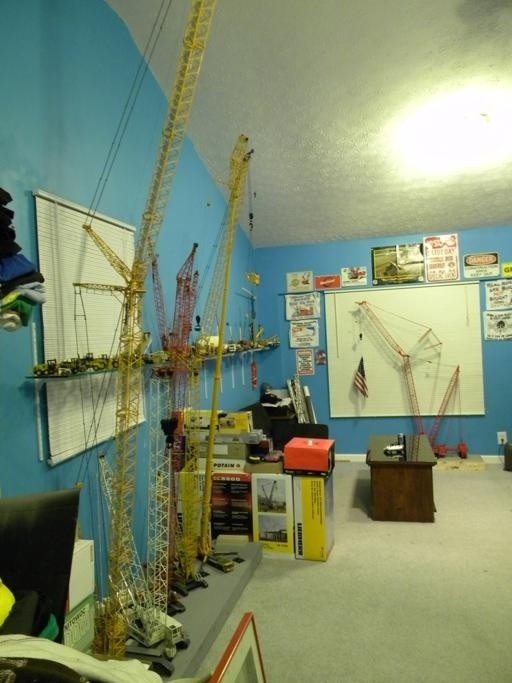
[{"x1": 354, "y1": 358, "x2": 368, "y2": 397}]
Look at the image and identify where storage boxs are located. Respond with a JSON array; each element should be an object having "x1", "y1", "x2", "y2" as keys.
[
  {"x1": 66, "y1": 538, "x2": 97, "y2": 611},
  {"x1": 279, "y1": 434, "x2": 337, "y2": 477},
  {"x1": 62, "y1": 597, "x2": 98, "y2": 655}
]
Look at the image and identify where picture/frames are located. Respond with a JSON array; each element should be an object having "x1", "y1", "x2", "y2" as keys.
[{"x1": 207, "y1": 611, "x2": 267, "y2": 683}]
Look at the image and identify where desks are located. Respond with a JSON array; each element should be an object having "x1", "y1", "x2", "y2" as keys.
[
  {"x1": 162, "y1": 445, "x2": 338, "y2": 560},
  {"x1": 365, "y1": 432, "x2": 437, "y2": 524}
]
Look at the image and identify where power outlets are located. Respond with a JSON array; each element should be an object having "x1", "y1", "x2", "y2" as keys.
[{"x1": 496, "y1": 429, "x2": 509, "y2": 446}]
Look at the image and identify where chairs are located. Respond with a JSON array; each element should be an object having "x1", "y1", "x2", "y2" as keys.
[{"x1": 271, "y1": 420, "x2": 328, "y2": 451}]
